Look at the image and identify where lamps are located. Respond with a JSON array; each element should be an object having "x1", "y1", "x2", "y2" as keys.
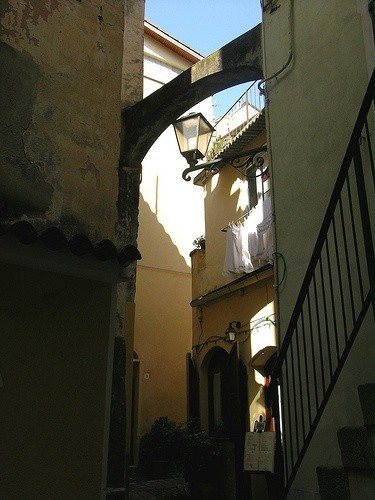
[
  {"x1": 172, "y1": 109, "x2": 269, "y2": 182},
  {"x1": 225, "y1": 320, "x2": 242, "y2": 343}
]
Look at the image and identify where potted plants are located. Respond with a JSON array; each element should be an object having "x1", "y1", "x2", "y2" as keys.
[{"x1": 192, "y1": 235, "x2": 205, "y2": 251}]
